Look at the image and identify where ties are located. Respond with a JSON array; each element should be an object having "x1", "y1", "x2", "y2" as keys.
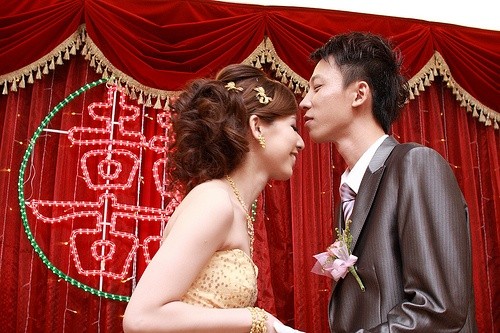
[{"x1": 338, "y1": 182, "x2": 358, "y2": 237}]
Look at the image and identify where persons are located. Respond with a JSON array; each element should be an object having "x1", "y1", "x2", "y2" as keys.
[
  {"x1": 272, "y1": 28, "x2": 479, "y2": 333},
  {"x1": 121, "y1": 60, "x2": 306, "y2": 333}
]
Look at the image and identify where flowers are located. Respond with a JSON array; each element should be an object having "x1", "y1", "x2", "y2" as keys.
[{"x1": 310, "y1": 218, "x2": 366, "y2": 292}]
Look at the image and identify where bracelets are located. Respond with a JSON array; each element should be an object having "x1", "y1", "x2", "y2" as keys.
[{"x1": 246, "y1": 306, "x2": 268, "y2": 333}]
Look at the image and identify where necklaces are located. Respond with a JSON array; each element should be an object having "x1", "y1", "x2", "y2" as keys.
[{"x1": 223, "y1": 172, "x2": 257, "y2": 261}]
